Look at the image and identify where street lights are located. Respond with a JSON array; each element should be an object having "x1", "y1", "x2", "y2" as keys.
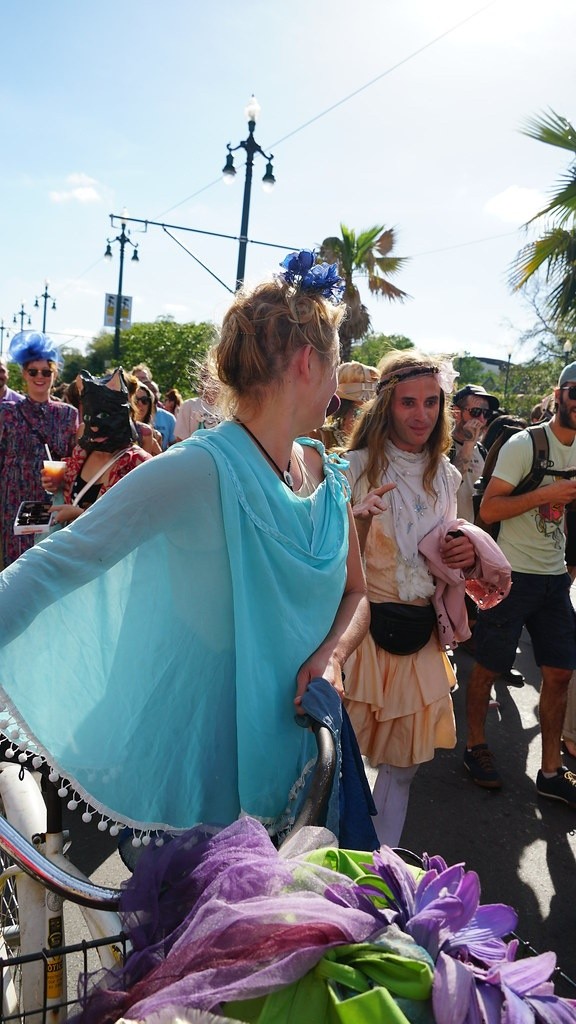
[
  {"x1": 32, "y1": 278, "x2": 57, "y2": 335},
  {"x1": 102, "y1": 206, "x2": 141, "y2": 366},
  {"x1": 219, "y1": 92, "x2": 277, "y2": 301},
  {"x1": 13, "y1": 299, "x2": 33, "y2": 332}
]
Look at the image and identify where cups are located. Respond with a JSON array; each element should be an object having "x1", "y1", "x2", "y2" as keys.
[{"x1": 43, "y1": 460, "x2": 67, "y2": 494}]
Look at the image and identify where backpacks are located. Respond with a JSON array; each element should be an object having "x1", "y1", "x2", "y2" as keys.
[{"x1": 472, "y1": 422, "x2": 547, "y2": 516}]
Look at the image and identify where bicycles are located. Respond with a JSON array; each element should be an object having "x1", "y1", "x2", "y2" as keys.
[{"x1": 0, "y1": 709, "x2": 336, "y2": 1024}]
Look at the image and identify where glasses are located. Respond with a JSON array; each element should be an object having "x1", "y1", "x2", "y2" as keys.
[
  {"x1": 25, "y1": 368, "x2": 53, "y2": 378},
  {"x1": 456, "y1": 405, "x2": 493, "y2": 419},
  {"x1": 559, "y1": 384, "x2": 576, "y2": 401},
  {"x1": 135, "y1": 395, "x2": 151, "y2": 405}
]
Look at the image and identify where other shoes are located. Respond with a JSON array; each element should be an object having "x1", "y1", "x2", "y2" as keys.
[
  {"x1": 505, "y1": 668, "x2": 525, "y2": 685},
  {"x1": 489, "y1": 700, "x2": 500, "y2": 707},
  {"x1": 558, "y1": 739, "x2": 576, "y2": 761}
]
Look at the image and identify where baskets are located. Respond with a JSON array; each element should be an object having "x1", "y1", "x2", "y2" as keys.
[{"x1": 0, "y1": 846, "x2": 574, "y2": 1024}]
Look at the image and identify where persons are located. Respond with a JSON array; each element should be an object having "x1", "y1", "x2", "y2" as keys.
[
  {"x1": 328, "y1": 353, "x2": 576, "y2": 854},
  {"x1": 0, "y1": 330, "x2": 233, "y2": 571},
  {"x1": 0, "y1": 286, "x2": 373, "y2": 904}
]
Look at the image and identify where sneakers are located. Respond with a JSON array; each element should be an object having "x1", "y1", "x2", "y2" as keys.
[
  {"x1": 536, "y1": 764, "x2": 576, "y2": 807},
  {"x1": 463, "y1": 742, "x2": 497, "y2": 787}
]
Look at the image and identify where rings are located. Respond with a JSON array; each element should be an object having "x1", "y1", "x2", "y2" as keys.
[
  {"x1": 52, "y1": 519, "x2": 58, "y2": 524},
  {"x1": 474, "y1": 422, "x2": 477, "y2": 426}
]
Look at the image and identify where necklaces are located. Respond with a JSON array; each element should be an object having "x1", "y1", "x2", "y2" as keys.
[{"x1": 236, "y1": 417, "x2": 294, "y2": 491}]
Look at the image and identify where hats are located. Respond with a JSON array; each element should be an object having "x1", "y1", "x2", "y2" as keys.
[
  {"x1": 559, "y1": 360, "x2": 576, "y2": 386},
  {"x1": 451, "y1": 383, "x2": 500, "y2": 412},
  {"x1": 335, "y1": 361, "x2": 377, "y2": 402}
]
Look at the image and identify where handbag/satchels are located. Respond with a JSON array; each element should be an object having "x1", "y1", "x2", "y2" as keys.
[{"x1": 366, "y1": 599, "x2": 436, "y2": 656}]
